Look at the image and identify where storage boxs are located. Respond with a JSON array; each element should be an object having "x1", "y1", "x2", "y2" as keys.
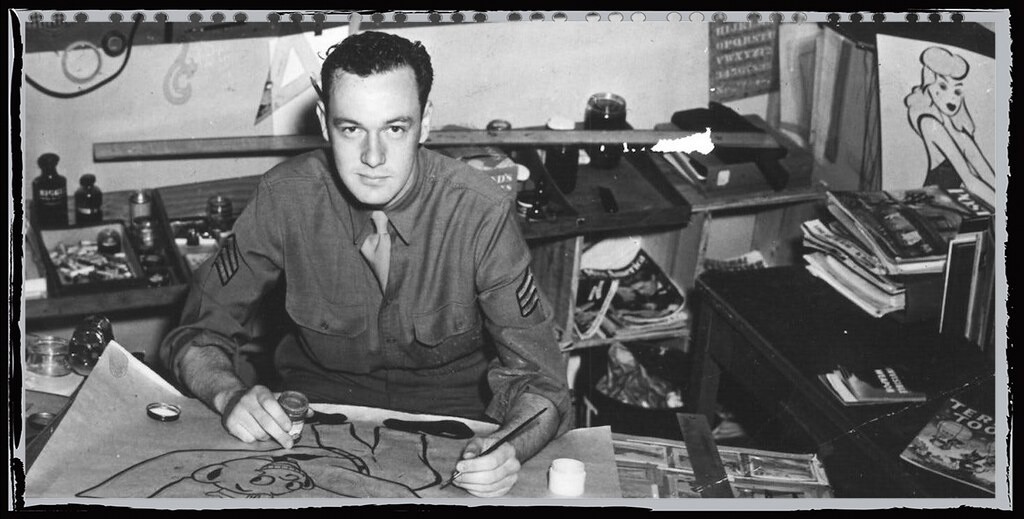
[
  {"x1": 443, "y1": 146, "x2": 518, "y2": 199},
  {"x1": 39, "y1": 219, "x2": 148, "y2": 298}
]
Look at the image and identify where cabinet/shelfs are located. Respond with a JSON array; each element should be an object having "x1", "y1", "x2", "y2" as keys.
[{"x1": 25, "y1": 113, "x2": 995, "y2": 509}]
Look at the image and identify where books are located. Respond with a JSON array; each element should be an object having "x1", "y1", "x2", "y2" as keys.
[{"x1": 800, "y1": 189, "x2": 995, "y2": 496}]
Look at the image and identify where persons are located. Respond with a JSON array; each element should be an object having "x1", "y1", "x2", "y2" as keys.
[{"x1": 161, "y1": 30, "x2": 573, "y2": 499}]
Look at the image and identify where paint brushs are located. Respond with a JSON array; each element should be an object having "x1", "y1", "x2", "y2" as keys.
[{"x1": 441, "y1": 407, "x2": 548, "y2": 486}]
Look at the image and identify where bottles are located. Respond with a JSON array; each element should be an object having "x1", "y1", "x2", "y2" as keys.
[
  {"x1": 274, "y1": 389, "x2": 308, "y2": 447},
  {"x1": 543, "y1": 116, "x2": 578, "y2": 193},
  {"x1": 29, "y1": 153, "x2": 103, "y2": 229},
  {"x1": 581, "y1": 92, "x2": 626, "y2": 168}
]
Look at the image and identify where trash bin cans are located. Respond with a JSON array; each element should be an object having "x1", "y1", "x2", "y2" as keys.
[{"x1": 584, "y1": 365, "x2": 691, "y2": 441}]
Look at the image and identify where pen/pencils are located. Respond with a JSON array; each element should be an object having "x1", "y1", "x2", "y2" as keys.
[{"x1": 310, "y1": 76, "x2": 324, "y2": 103}]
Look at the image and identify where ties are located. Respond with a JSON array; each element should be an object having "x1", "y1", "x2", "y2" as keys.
[{"x1": 360, "y1": 211, "x2": 391, "y2": 295}]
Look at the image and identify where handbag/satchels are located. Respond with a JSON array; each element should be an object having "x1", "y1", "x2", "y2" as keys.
[
  {"x1": 595, "y1": 341, "x2": 684, "y2": 408},
  {"x1": 573, "y1": 235, "x2": 685, "y2": 340}
]
